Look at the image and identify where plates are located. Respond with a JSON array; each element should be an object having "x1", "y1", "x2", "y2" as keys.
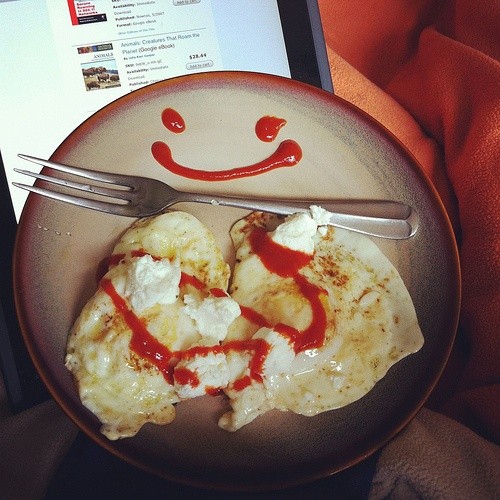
[{"x1": 11, "y1": 70, "x2": 463, "y2": 494}]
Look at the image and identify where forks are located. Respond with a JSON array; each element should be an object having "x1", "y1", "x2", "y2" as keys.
[{"x1": 10, "y1": 151, "x2": 423, "y2": 241}]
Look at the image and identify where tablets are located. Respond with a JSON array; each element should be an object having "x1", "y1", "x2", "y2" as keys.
[{"x1": 0, "y1": 1, "x2": 335, "y2": 415}]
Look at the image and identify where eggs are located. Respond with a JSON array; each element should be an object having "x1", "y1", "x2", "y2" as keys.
[
  {"x1": 218, "y1": 210, "x2": 425, "y2": 433},
  {"x1": 64, "y1": 211, "x2": 241, "y2": 441}
]
